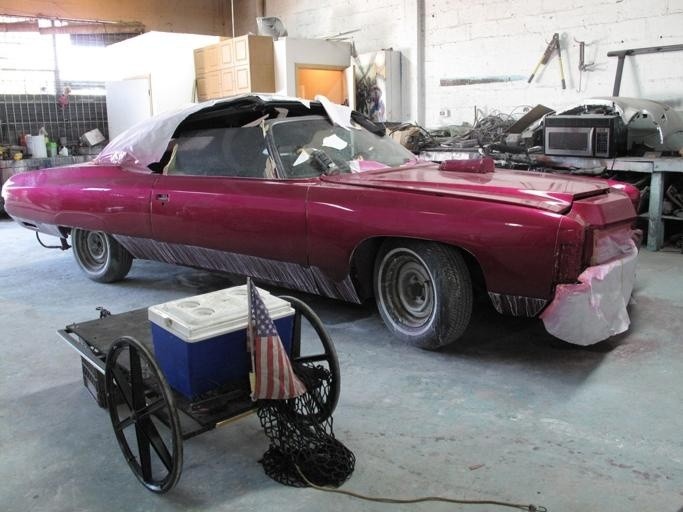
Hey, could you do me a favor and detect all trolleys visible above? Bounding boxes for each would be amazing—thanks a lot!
[51,282,347,497]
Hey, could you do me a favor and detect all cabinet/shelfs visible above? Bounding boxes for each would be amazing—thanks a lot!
[193,35,275,102]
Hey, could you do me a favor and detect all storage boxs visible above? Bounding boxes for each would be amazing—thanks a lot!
[148,285,296,401]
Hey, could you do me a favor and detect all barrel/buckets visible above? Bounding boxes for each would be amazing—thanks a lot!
[26,135,47,158]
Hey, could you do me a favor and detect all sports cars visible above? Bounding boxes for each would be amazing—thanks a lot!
[0,90,654,358]
